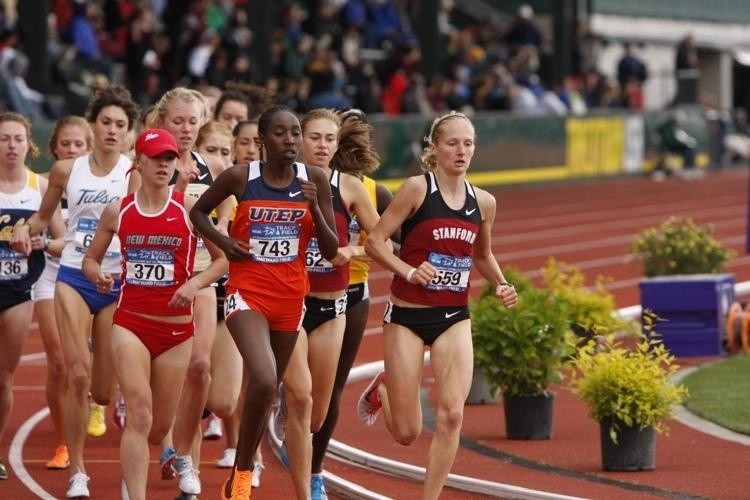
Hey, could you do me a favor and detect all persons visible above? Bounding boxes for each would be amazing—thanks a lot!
[1,1,650,126]
[670,36,701,109]
[652,113,699,181]
[1,79,517,499]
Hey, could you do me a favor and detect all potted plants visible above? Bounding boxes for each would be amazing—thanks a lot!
[469,268,577,439]
[560,307,688,472]
[465,294,501,402]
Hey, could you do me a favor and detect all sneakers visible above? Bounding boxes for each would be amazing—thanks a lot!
[310,472,328,500]
[173,491,198,500]
[0,461,8,479]
[159,448,176,480]
[216,448,237,468]
[112,394,126,430]
[204,416,223,438]
[170,455,202,494]
[279,443,289,468]
[85,402,107,437]
[121,480,130,500]
[356,370,386,426]
[221,465,251,500]
[45,444,70,468]
[251,459,266,487]
[65,472,91,499]
[273,382,287,441]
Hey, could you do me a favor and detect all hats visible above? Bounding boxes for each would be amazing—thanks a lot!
[134,128,180,159]
